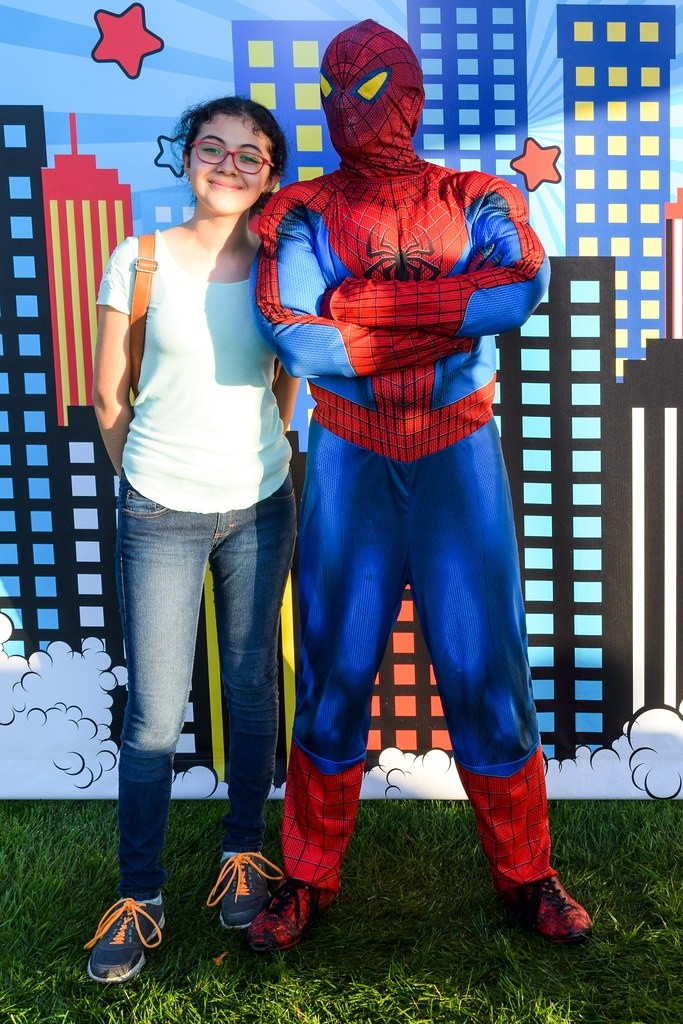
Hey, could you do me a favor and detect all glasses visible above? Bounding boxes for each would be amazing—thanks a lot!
[189,139,276,174]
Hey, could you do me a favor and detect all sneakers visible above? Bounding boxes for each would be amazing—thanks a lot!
[247,871,338,951]
[205,852,284,929]
[84,890,166,983]
[503,873,592,942]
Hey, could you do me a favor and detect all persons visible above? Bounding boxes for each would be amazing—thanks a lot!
[243,17,594,951]
[82,97,301,980]
